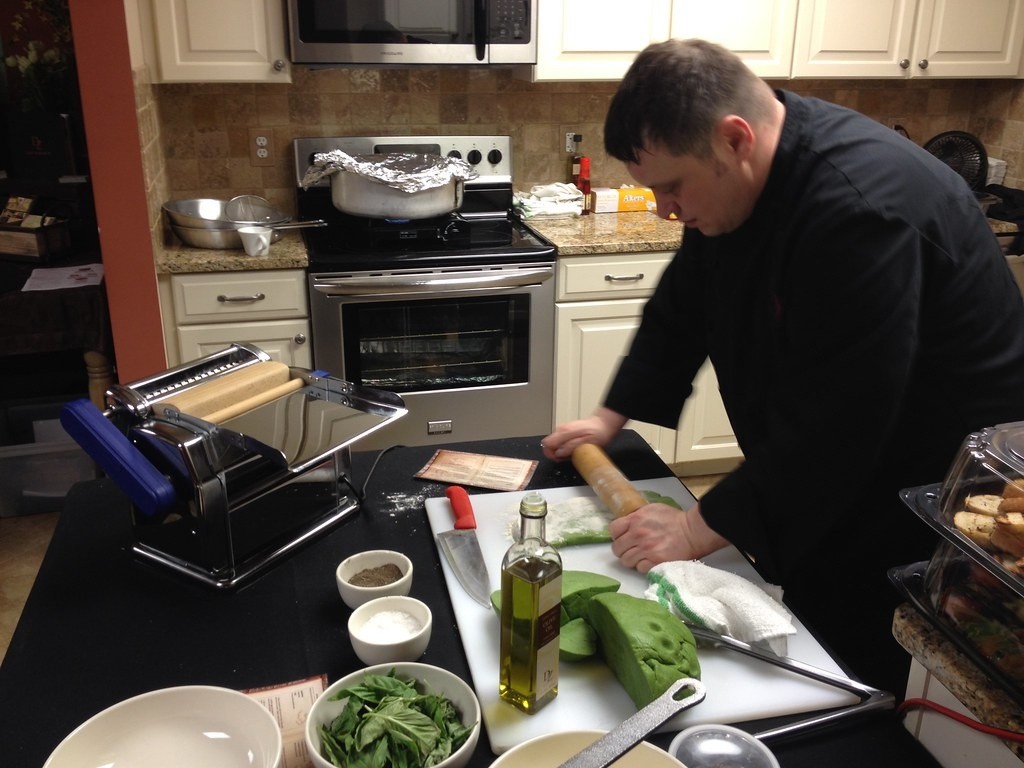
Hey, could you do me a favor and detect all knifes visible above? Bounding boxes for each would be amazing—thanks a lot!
[436,485,493,609]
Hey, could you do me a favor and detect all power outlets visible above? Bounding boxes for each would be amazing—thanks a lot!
[888,118,905,136]
[249,128,275,167]
[559,125,579,159]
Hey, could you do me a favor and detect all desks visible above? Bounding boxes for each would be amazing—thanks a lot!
[0,248,113,412]
[1,429,942,767]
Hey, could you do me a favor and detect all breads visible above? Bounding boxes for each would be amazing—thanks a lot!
[955,480,1024,582]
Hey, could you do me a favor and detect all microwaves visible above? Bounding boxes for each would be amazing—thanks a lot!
[285,0,539,72]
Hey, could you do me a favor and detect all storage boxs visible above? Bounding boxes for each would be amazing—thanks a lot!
[0,394,101,517]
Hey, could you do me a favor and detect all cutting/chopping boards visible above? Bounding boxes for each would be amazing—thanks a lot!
[423,475,864,754]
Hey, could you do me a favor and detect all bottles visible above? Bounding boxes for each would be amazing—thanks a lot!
[566,134,585,184]
[576,157,591,215]
[499,492,564,715]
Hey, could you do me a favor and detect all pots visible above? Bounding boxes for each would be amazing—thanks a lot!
[162,199,328,248]
[330,166,455,216]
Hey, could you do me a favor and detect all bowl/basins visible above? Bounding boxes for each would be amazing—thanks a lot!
[347,595,433,667]
[42,684,284,768]
[488,729,687,768]
[668,722,781,768]
[335,549,413,610]
[305,662,482,768]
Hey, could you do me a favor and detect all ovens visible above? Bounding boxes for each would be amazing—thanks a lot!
[307,262,555,453]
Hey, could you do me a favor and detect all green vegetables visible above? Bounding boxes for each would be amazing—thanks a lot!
[957,620,1021,661]
[320,667,478,768]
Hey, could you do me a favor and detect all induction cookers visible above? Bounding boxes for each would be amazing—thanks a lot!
[291,134,559,278]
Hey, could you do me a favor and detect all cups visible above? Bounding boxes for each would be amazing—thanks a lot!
[237,226,273,257]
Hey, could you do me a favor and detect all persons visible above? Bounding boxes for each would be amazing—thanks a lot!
[542,40,1023,706]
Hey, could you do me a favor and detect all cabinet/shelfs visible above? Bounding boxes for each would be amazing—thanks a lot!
[171,270,312,371]
[512,0,799,80]
[137,0,292,84]
[791,0,1024,79]
[552,250,745,479]
